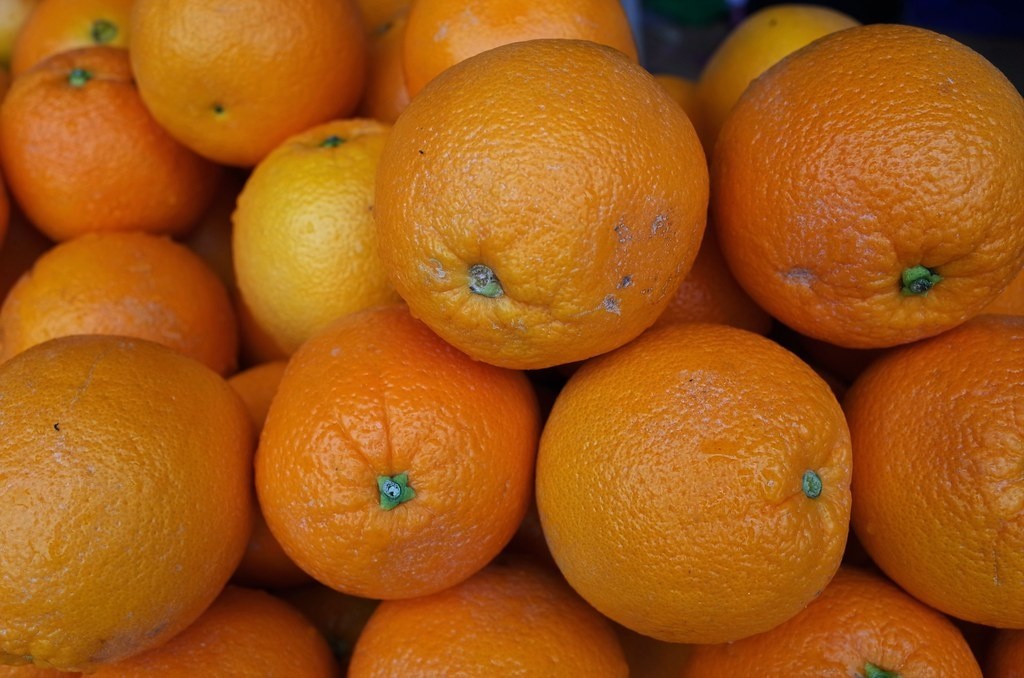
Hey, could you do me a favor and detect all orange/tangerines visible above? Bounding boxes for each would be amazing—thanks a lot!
[0,0,1024,678]
